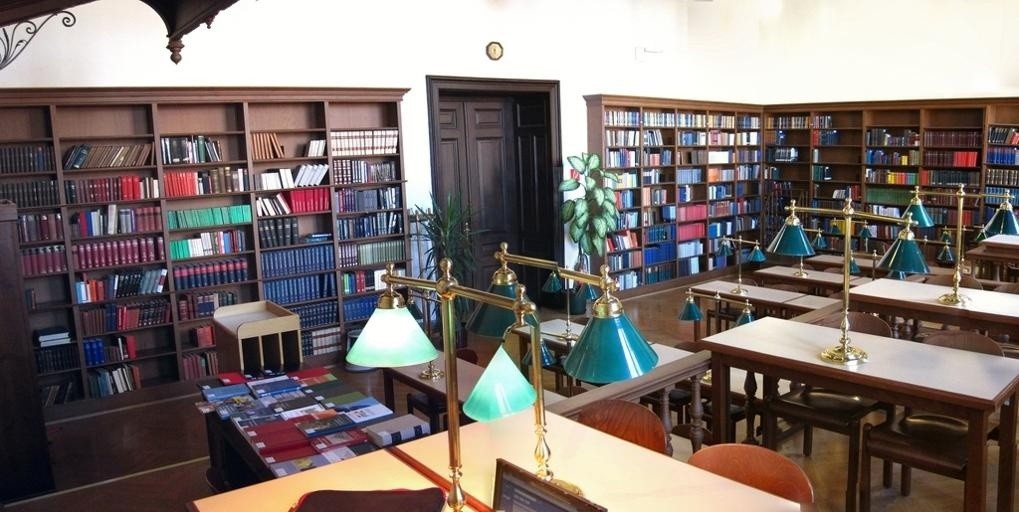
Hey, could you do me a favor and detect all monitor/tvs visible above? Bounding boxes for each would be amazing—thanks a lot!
[493,458,607,512]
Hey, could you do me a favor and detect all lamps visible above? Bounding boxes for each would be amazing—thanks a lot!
[466,243,659,384]
[764,183,931,364]
[716,235,766,295]
[899,183,1018,306]
[679,287,755,332]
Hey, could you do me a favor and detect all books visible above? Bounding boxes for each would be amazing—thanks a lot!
[865,125,1019,241]
[1,126,407,406]
[199,366,431,481]
[603,110,862,293]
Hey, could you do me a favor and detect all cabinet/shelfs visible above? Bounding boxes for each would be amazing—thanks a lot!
[1,88,416,425]
